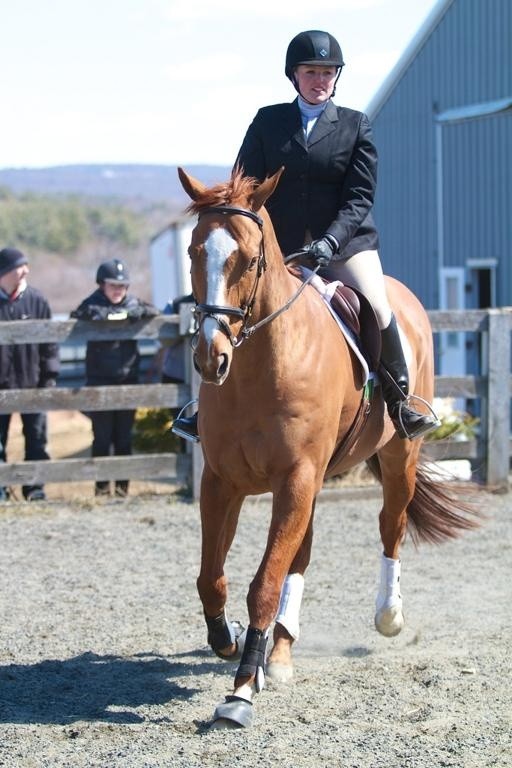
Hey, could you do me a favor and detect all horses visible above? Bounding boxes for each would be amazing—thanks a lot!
[177,156,497,732]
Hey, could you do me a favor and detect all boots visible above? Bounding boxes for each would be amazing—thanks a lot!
[173,411,198,436]
[378,312,430,440]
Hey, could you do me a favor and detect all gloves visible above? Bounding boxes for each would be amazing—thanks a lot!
[302,239,334,268]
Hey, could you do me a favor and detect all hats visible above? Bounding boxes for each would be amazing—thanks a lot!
[1,249,27,275]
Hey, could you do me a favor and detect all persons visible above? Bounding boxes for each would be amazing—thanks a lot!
[68,258,160,505]
[0,247,60,504]
[168,31,436,441]
[158,293,201,503]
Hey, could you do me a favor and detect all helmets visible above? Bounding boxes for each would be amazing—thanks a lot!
[97,261,130,288]
[286,31,344,78]
